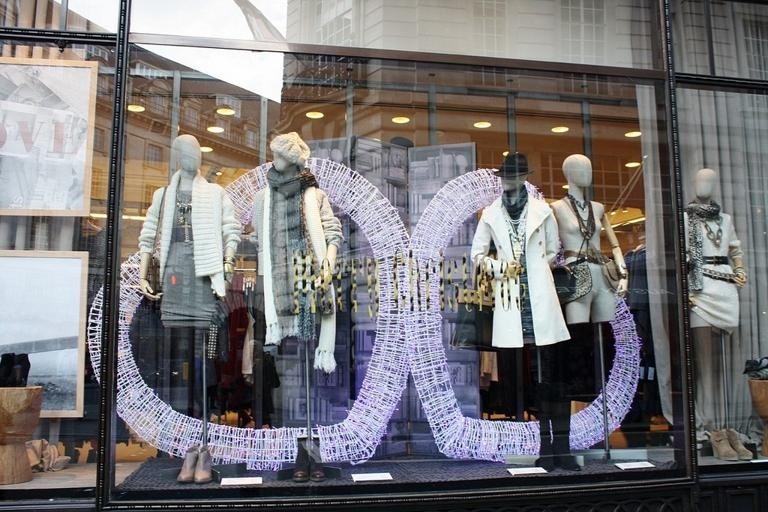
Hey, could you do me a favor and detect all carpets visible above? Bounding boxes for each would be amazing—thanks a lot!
[117,458,676,493]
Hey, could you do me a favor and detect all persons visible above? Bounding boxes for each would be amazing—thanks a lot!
[684,169,754,462]
[139,135,243,326]
[471,150,626,351]
[252,133,339,314]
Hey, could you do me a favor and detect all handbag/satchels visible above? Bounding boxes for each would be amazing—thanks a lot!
[602,259,618,292]
[552,258,592,305]
[147,258,160,296]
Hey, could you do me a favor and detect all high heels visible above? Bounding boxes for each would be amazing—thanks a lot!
[710,428,753,461]
[1,353,30,386]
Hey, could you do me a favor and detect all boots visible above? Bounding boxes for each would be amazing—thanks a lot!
[535,383,580,471]
[294,437,325,482]
[177,447,212,482]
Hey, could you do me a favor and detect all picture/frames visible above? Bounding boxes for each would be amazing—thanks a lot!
[0,56,100,217]
[2,249,90,420]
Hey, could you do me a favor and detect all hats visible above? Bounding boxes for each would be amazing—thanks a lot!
[269,132,310,165]
[493,152,534,178]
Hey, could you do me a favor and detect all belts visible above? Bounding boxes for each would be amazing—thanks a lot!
[702,256,728,264]
[565,250,595,261]
